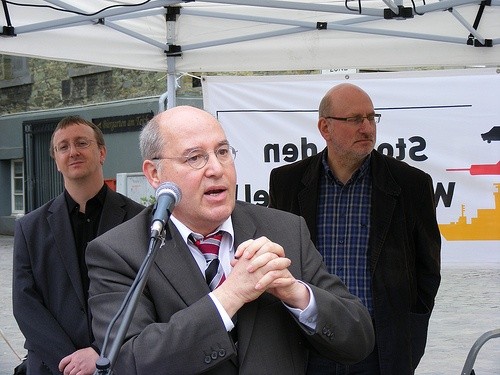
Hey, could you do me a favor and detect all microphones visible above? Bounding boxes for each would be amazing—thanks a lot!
[149,182,182,242]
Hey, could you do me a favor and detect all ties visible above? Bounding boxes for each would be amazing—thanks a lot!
[187,230,226,292]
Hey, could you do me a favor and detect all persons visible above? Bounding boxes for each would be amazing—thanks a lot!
[268,83,442,375]
[12,116,147,375]
[85,105,375,374]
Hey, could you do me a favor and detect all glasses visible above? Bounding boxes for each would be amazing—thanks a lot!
[53,139,105,153]
[151,144,238,169]
[324,114,382,125]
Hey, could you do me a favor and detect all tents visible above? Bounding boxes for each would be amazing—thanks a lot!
[0,0,499,110]
